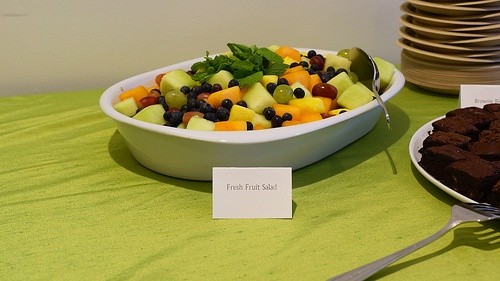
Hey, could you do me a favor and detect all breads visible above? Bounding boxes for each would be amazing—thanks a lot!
[417,104,500,206]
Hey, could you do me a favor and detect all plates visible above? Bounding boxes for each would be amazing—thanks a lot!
[100,47,405,181]
[408,106,499,220]
[395,1,500,94]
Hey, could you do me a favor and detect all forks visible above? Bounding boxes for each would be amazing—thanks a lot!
[326,202,500,281]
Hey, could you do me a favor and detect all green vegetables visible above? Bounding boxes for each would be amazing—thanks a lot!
[193,42,289,90]
